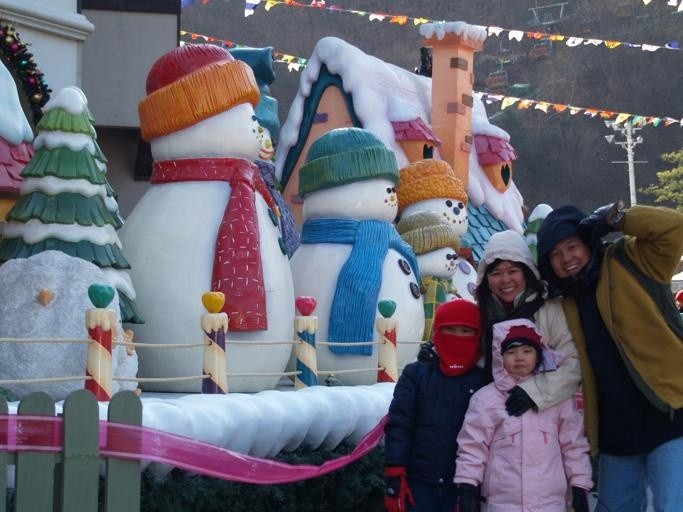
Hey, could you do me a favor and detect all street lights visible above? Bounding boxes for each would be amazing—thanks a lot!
[601,117,648,207]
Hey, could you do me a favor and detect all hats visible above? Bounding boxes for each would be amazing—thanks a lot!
[501,325,542,356]
[430,300,482,377]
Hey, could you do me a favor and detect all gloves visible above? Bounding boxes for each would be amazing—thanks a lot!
[505,385,534,416]
[385,467,415,512]
[458,483,481,512]
[571,486,589,512]
[579,202,625,236]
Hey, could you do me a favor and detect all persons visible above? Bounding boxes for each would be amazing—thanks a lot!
[674,290,683,319]
[417,230,582,416]
[455,319,593,512]
[537,201,683,511]
[383,299,494,512]
